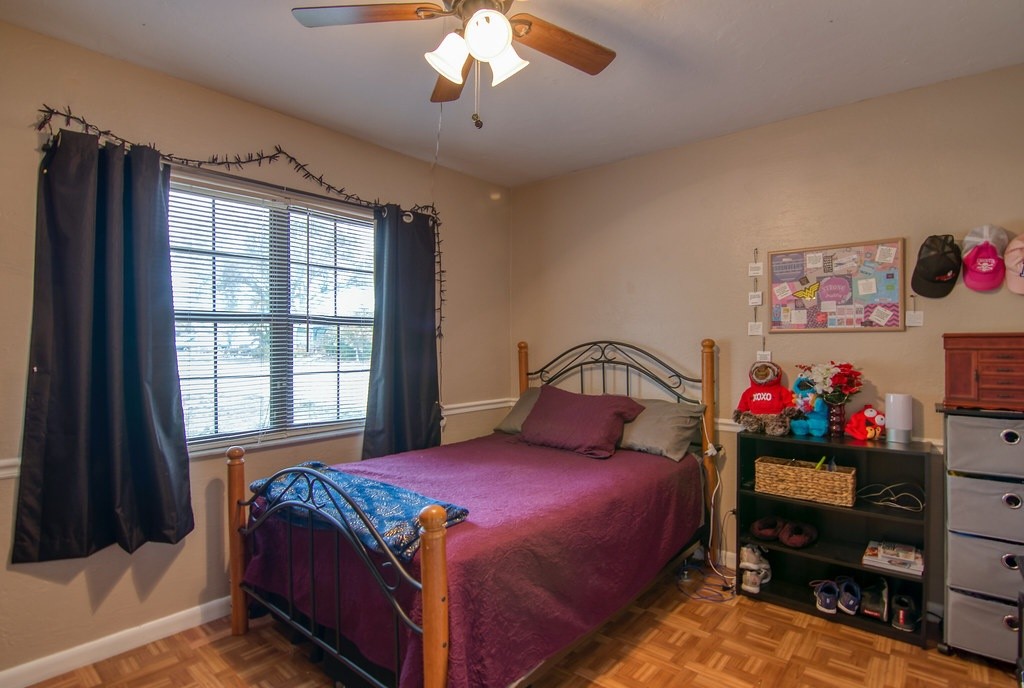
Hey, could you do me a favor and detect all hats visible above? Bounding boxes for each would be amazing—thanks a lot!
[911,234,961,298]
[961,224,1008,290]
[1003,235,1024,294]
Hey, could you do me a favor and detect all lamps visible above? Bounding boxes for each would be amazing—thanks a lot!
[424,0,530,129]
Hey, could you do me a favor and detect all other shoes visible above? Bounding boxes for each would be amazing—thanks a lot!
[752,518,784,540]
[779,521,818,548]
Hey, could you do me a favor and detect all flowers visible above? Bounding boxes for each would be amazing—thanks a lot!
[794,360,864,405]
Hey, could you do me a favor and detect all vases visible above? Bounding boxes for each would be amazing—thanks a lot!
[824,395,850,438]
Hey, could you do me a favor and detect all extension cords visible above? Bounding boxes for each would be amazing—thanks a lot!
[708,443,717,456]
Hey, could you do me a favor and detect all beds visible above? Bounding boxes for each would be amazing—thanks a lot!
[227,338,719,688]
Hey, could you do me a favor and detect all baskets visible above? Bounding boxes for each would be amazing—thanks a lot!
[754,457,855,508]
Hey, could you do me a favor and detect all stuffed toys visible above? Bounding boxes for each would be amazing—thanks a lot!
[845,405,886,440]
[731,361,796,435]
[790,372,829,435]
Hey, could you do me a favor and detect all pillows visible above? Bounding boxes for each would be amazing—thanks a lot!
[617,398,707,462]
[494,387,540,435]
[506,384,645,459]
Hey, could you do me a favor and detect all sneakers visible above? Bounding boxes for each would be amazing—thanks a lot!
[835,573,860,615]
[741,572,761,594]
[809,579,839,613]
[740,546,770,570]
[890,593,916,632]
[860,577,889,622]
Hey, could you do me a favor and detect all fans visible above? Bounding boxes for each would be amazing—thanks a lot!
[291,0,616,102]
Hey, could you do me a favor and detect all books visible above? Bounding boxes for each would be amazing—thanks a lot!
[862,540,924,575]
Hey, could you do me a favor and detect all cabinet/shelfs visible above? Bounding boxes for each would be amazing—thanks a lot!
[941,332,1024,412]
[735,428,933,651]
[934,402,1024,685]
